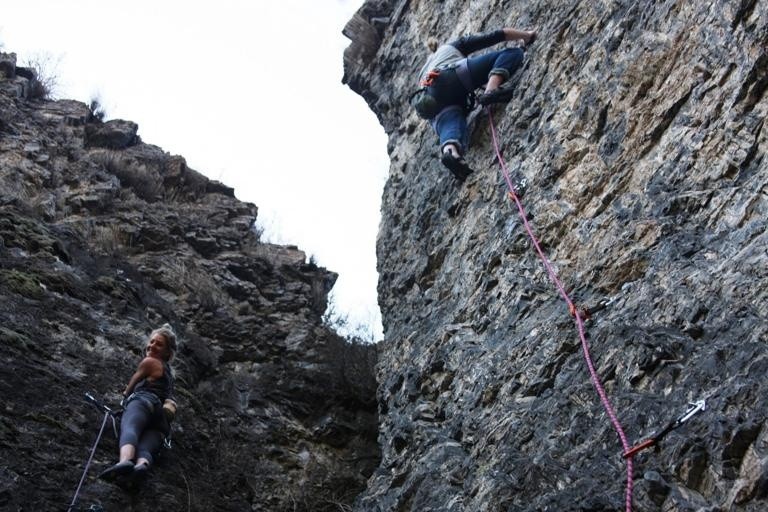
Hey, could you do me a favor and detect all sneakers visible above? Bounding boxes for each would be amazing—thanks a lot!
[477,86,515,106]
[440,147,473,181]
[121,461,149,485]
[98,458,135,482]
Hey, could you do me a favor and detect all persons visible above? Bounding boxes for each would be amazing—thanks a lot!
[417,25,540,181]
[97,322,178,488]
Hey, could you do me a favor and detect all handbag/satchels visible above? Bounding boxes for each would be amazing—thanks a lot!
[415,95,437,120]
